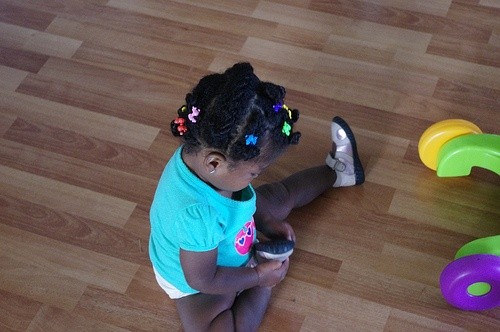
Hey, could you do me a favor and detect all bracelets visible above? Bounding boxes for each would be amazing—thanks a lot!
[253,265,262,288]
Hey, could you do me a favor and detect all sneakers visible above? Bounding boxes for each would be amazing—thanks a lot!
[327,117,366,188]
[255,239,294,264]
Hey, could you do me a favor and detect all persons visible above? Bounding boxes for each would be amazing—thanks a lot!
[148,60,365,332]
